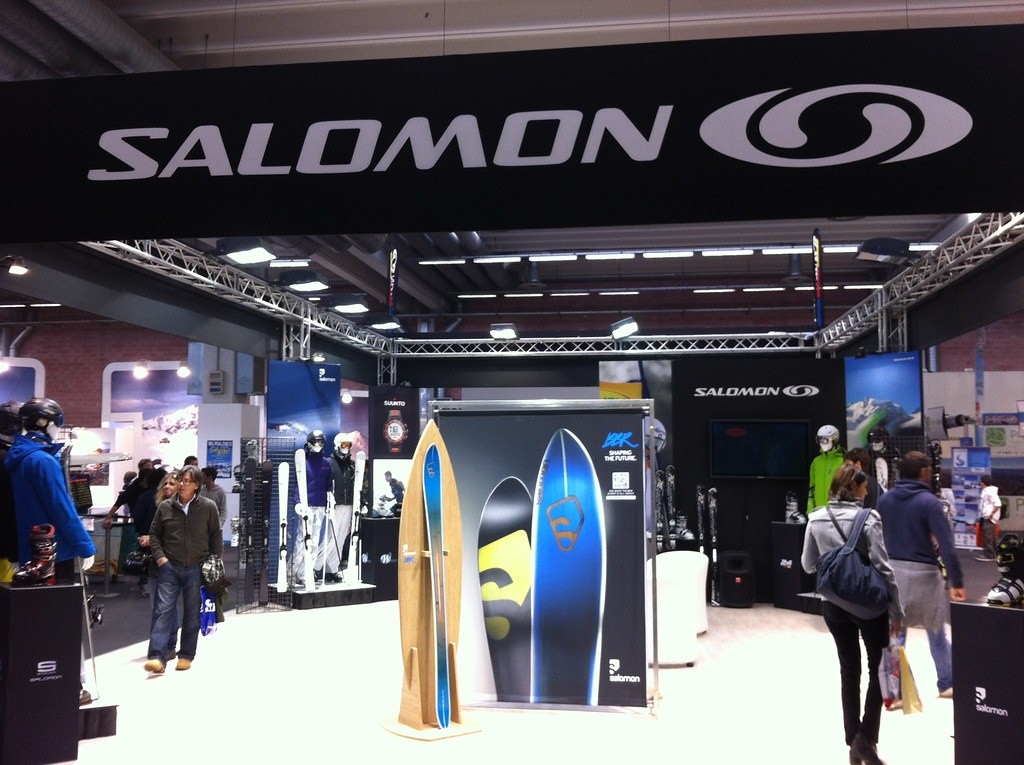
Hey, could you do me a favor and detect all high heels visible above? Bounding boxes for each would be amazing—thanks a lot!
[849,733,887,765]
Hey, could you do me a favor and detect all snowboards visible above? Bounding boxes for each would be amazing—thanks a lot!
[476,475,534,702]
[532,426,607,705]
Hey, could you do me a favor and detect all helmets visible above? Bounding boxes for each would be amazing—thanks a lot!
[0,400,24,434]
[867,427,889,440]
[818,425,840,439]
[20,397,64,431]
[334,432,354,444]
[306,430,326,443]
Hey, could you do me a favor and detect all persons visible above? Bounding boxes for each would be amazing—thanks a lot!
[383,469,406,516]
[286,429,333,588]
[873,452,967,704]
[806,425,850,515]
[313,431,355,582]
[0,396,96,585]
[939,471,958,527]
[845,446,884,510]
[798,463,908,765]
[100,453,227,672]
[865,427,903,493]
[975,475,1001,561]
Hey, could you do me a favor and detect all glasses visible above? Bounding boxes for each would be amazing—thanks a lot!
[342,441,353,449]
[816,436,830,444]
[175,478,197,486]
[868,436,883,444]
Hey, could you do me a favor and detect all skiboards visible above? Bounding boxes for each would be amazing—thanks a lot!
[655,463,676,554]
[421,443,452,730]
[241,456,273,606]
[695,484,721,607]
[888,439,944,501]
[276,449,316,594]
[345,449,367,584]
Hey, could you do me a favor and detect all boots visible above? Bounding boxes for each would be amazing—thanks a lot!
[12,523,57,586]
[785,493,807,524]
[675,514,694,540]
[986,533,1024,604]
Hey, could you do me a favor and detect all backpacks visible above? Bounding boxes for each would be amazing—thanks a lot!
[999,497,1010,520]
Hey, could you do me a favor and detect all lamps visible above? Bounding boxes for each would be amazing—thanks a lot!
[313,351,352,406]
[207,237,942,341]
[0,254,27,275]
[134,356,190,380]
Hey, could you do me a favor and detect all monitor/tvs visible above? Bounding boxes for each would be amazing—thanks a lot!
[708,418,811,479]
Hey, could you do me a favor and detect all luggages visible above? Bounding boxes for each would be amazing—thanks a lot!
[717,549,756,608]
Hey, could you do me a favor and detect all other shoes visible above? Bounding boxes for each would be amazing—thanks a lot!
[313,568,344,582]
[974,552,997,561]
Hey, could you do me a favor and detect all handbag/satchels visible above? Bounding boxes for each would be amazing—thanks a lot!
[815,508,898,620]
[878,632,924,715]
[121,543,148,571]
[200,585,216,636]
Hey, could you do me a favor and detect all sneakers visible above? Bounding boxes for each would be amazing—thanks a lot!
[144,659,165,673]
[176,658,191,670]
